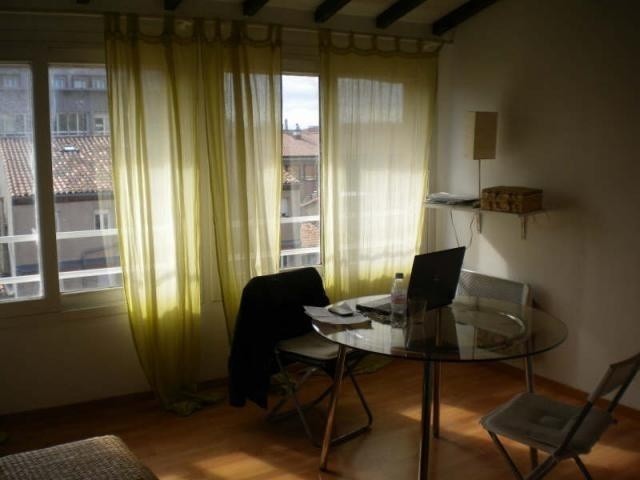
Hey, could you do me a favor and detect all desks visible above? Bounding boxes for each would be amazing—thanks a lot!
[312,294,567,480]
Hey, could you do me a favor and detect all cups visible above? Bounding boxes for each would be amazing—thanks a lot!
[409,296,428,324]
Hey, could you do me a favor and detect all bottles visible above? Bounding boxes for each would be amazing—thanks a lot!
[390,272,409,328]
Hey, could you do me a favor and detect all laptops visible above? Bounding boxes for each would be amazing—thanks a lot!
[355,246,465,320]
[363,307,462,360]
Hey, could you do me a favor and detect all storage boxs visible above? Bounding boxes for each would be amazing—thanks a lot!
[481,186,543,213]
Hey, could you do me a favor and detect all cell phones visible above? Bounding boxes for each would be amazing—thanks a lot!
[328,307,353,317]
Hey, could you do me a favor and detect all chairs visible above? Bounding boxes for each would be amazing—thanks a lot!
[250,267,373,450]
[478,353,640,480]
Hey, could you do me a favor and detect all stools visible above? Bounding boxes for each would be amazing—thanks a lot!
[0,434,159,480]
